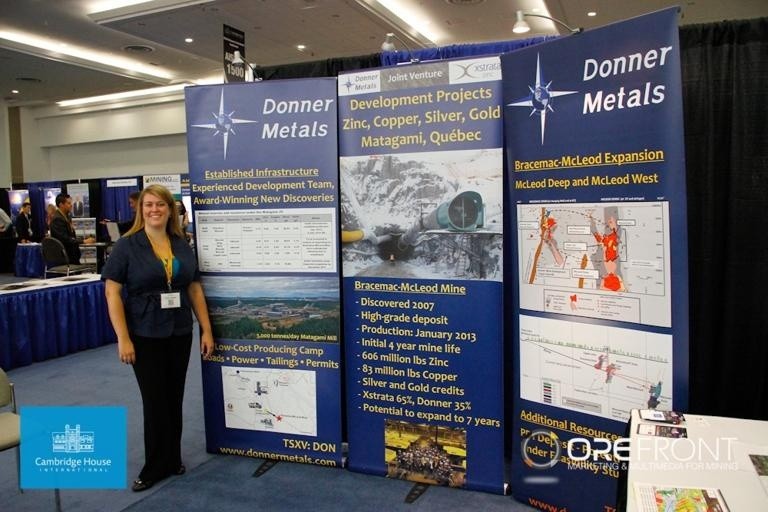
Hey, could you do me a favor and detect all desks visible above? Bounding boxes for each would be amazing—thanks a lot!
[14,242,43,278]
[0,272,119,371]
[78,242,111,273]
[624,408,768,512]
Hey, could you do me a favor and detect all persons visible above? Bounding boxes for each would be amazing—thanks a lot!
[0,208,13,233]
[102,183,215,494]
[174,200,190,244]
[46,204,56,230]
[16,203,39,277]
[50,193,96,276]
[99,191,141,236]
[73,192,84,216]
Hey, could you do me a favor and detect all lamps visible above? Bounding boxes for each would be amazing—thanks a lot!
[512,10,583,36]
[231,49,263,83]
[380,32,420,64]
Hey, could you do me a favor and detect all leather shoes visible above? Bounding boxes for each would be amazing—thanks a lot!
[131,464,187,492]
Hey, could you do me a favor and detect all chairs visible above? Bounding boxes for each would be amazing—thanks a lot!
[42,237,95,280]
[0,368,61,512]
